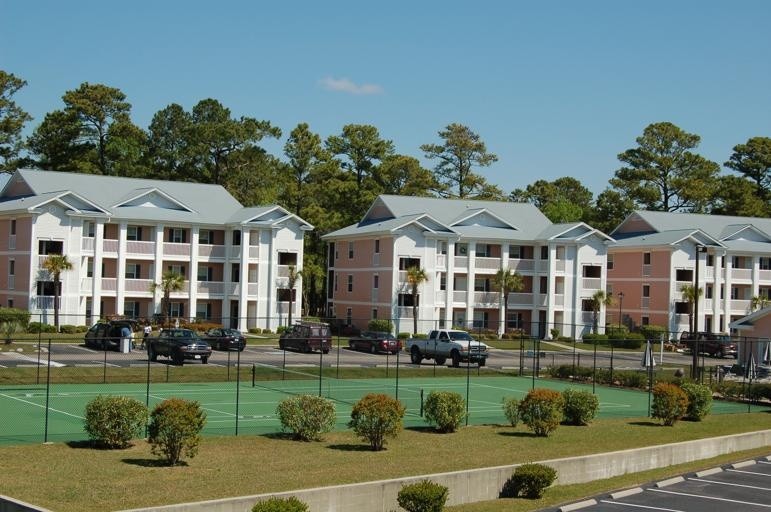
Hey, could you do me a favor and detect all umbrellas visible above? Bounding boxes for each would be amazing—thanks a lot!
[641,340,657,375]
[764,339,771,366]
[747,353,758,382]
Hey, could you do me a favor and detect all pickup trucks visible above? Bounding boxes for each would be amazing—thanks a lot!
[144,326,212,365]
[404,330,488,368]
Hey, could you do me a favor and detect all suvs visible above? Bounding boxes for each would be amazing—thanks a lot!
[83,320,139,352]
[680,329,738,359]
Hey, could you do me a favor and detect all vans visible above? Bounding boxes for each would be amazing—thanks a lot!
[280,319,331,354]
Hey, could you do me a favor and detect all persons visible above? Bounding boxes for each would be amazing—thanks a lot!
[141,320,153,350]
[121,324,132,354]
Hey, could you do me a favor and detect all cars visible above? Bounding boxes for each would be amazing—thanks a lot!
[347,330,400,355]
[198,326,246,352]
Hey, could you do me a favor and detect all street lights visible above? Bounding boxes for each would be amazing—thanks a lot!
[616,290,624,333]
[690,243,726,381]
